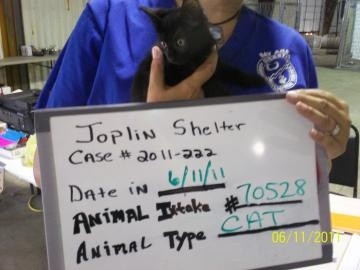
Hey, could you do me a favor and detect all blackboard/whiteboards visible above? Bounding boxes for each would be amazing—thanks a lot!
[32,89,336,270]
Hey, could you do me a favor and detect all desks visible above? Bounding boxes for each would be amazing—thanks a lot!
[0,50,63,86]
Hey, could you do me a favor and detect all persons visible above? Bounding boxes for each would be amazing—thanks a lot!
[26,0,351,191]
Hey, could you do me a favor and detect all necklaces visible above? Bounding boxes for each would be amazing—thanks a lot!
[204,9,240,42]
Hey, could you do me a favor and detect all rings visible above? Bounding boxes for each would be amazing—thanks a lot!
[330,124,341,137]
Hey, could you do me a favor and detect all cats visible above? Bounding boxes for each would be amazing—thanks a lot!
[131,0,267,102]
